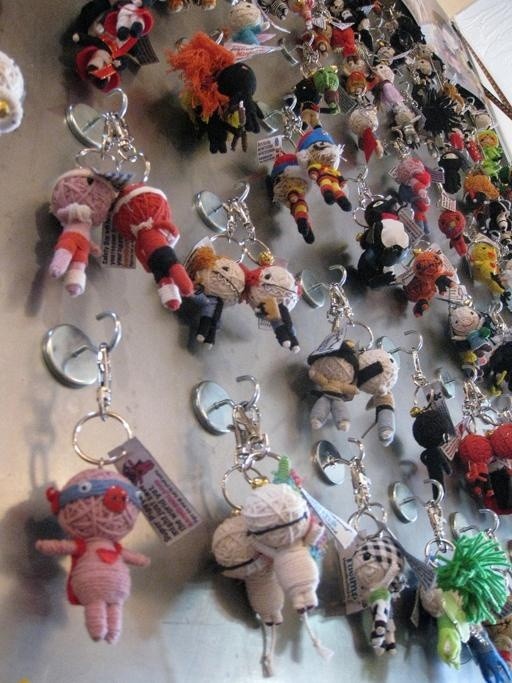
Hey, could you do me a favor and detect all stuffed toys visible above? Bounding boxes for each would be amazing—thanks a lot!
[1,1,510,682]
[0,0,510,683]
